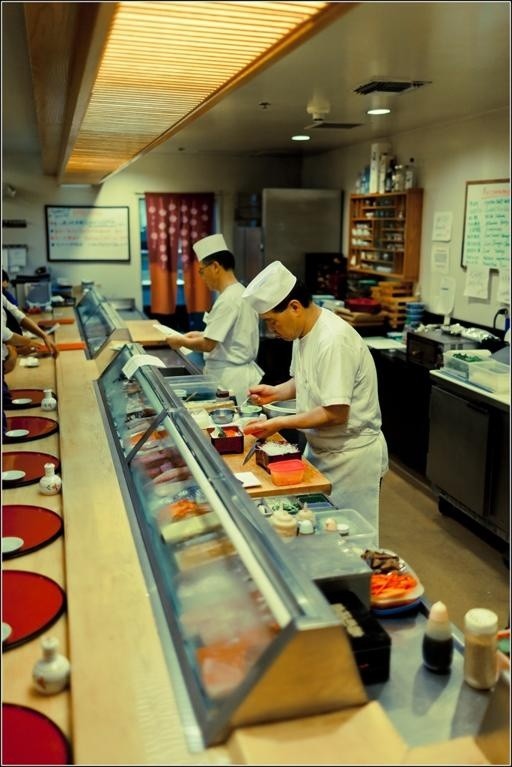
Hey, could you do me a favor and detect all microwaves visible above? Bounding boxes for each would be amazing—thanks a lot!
[406,331,478,371]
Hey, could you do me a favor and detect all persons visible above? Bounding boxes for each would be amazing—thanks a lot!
[3,269,21,307]
[243,278,389,548]
[15,341,41,355]
[1,343,18,434]
[165,249,265,402]
[2,292,60,358]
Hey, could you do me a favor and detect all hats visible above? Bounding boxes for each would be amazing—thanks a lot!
[192,232,240,263]
[240,259,300,315]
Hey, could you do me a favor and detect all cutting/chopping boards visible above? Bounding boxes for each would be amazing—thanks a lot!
[125,319,168,346]
[201,419,332,498]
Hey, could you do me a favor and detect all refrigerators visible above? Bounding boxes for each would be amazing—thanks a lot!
[233,188,344,287]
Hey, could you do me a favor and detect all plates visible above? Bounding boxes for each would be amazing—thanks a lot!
[2,620,12,643]
[2,536,24,553]
[232,471,261,489]
[12,398,32,406]
[357,548,424,616]
[5,429,30,438]
[2,470,26,481]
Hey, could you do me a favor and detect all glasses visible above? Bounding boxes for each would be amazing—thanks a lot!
[198,259,215,273]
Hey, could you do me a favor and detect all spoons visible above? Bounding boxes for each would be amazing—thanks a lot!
[232,395,251,408]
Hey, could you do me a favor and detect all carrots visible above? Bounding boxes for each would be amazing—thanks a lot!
[372,571,416,596]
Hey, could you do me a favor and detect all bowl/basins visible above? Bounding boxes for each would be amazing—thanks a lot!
[405,302,425,326]
[236,405,263,418]
[387,331,404,342]
[345,298,381,315]
[209,408,236,424]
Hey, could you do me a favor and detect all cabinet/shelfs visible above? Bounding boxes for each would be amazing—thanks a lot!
[345,187,423,282]
[426,384,509,569]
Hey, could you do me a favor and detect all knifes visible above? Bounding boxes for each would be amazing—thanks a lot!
[243,437,266,466]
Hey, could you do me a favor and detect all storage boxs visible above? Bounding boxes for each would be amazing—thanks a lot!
[442,350,510,393]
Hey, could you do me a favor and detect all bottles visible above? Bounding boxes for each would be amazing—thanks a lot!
[384,155,416,192]
[269,501,349,538]
[39,463,62,496]
[215,391,229,403]
[31,636,69,696]
[355,172,365,194]
[41,389,57,411]
[462,607,500,691]
[421,600,455,674]
